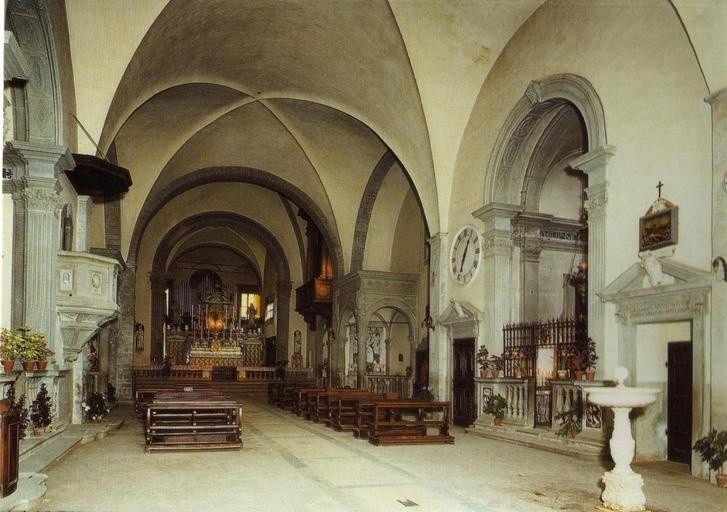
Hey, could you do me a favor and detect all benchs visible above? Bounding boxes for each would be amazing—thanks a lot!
[135,381,243,454]
[267,380,457,446]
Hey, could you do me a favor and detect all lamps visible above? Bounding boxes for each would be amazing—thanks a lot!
[134,322,144,352]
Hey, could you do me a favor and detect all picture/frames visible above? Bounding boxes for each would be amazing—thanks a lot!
[637,207,677,251]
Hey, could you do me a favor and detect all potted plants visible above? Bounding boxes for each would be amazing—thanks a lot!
[476,337,600,383]
[693,427,726,489]
[1,327,52,372]
[1,381,117,439]
[483,392,507,426]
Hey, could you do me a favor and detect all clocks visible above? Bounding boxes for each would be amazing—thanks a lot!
[447,224,482,287]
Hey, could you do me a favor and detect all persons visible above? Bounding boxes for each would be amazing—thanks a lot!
[246,304,258,335]
[171,300,183,332]
[136,325,144,352]
[294,329,301,354]
